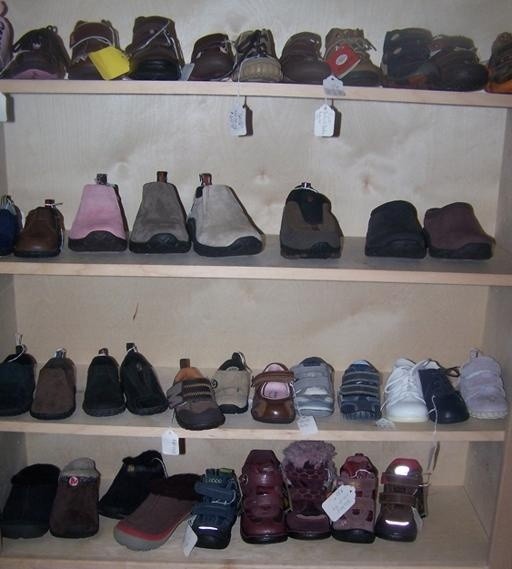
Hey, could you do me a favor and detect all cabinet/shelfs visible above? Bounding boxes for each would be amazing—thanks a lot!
[0,78,512,569]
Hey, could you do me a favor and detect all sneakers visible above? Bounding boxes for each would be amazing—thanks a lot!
[383,29,432,93]
[132,169,192,257]
[66,169,130,257]
[236,29,279,81]
[323,26,382,86]
[195,171,264,257]
[0,18,70,82]
[69,16,124,80]
[432,31,487,92]
[279,181,343,260]
[488,31,511,92]
[1,440,430,550]
[365,198,423,260]
[0,338,510,431]
[281,28,330,81]
[423,202,498,262]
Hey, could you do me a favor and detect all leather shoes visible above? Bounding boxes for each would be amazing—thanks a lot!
[189,34,235,82]
[129,17,185,80]
[0,193,65,259]
